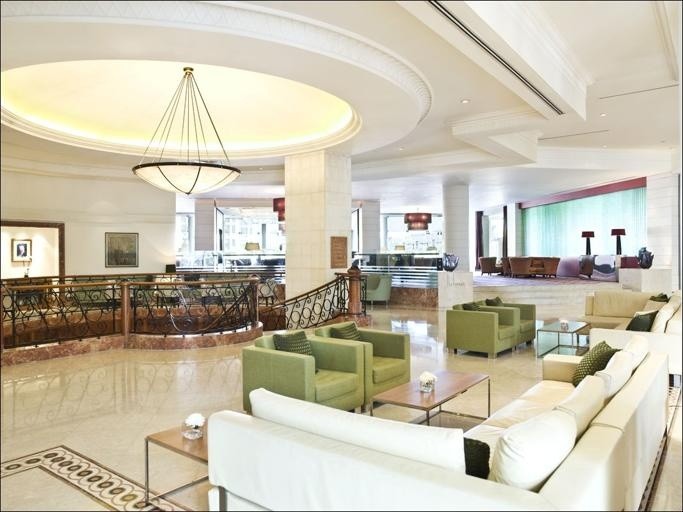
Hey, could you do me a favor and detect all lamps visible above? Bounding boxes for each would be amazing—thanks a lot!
[133,67,241,196]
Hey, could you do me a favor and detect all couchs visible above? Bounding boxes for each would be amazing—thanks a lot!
[208,350,670,511]
[361,275,392,304]
[446,305,520,359]
[530,256,560,278]
[478,256,503,276]
[585,289,682,375]
[501,257,512,277]
[315,321,410,412]
[481,298,535,350]
[242,328,365,412]
[509,257,536,278]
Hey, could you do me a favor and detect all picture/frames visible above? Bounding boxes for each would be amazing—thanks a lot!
[11,239,32,262]
[105,232,139,268]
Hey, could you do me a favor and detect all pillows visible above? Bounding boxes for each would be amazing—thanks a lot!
[486,297,503,307]
[273,332,317,373]
[249,388,464,471]
[597,352,634,392]
[464,437,490,480]
[626,311,657,332]
[330,323,361,340]
[490,410,578,490]
[572,342,621,387]
[463,302,481,310]
[643,293,670,311]
[555,376,608,438]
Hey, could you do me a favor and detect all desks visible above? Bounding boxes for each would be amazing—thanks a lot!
[373,371,490,426]
[144,418,208,510]
[537,322,590,358]
